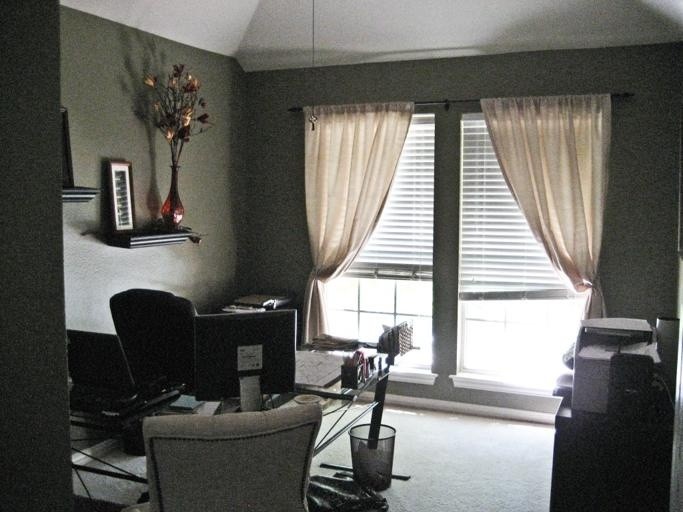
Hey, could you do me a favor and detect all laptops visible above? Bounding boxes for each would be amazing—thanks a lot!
[65,330,185,392]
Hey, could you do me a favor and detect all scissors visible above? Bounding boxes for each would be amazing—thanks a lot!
[345,356,355,367]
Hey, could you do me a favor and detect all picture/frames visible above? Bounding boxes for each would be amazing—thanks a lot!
[108,160,135,232]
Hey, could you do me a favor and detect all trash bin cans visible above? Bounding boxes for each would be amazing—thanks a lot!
[348,423,397,492]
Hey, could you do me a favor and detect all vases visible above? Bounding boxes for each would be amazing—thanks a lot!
[160,166,184,226]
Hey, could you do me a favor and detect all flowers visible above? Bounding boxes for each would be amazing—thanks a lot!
[139,65,216,212]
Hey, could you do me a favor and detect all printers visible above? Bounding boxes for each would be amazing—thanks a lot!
[552,318,652,397]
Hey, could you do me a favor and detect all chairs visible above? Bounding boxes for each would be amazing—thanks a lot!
[142,402,321,512]
[108,289,201,392]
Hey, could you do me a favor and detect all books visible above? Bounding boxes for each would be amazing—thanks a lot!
[295,349,350,388]
[162,398,222,416]
[169,393,206,415]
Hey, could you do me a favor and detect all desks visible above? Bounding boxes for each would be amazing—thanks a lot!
[288,334,420,446]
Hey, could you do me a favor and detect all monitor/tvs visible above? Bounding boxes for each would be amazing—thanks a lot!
[192,309,298,412]
